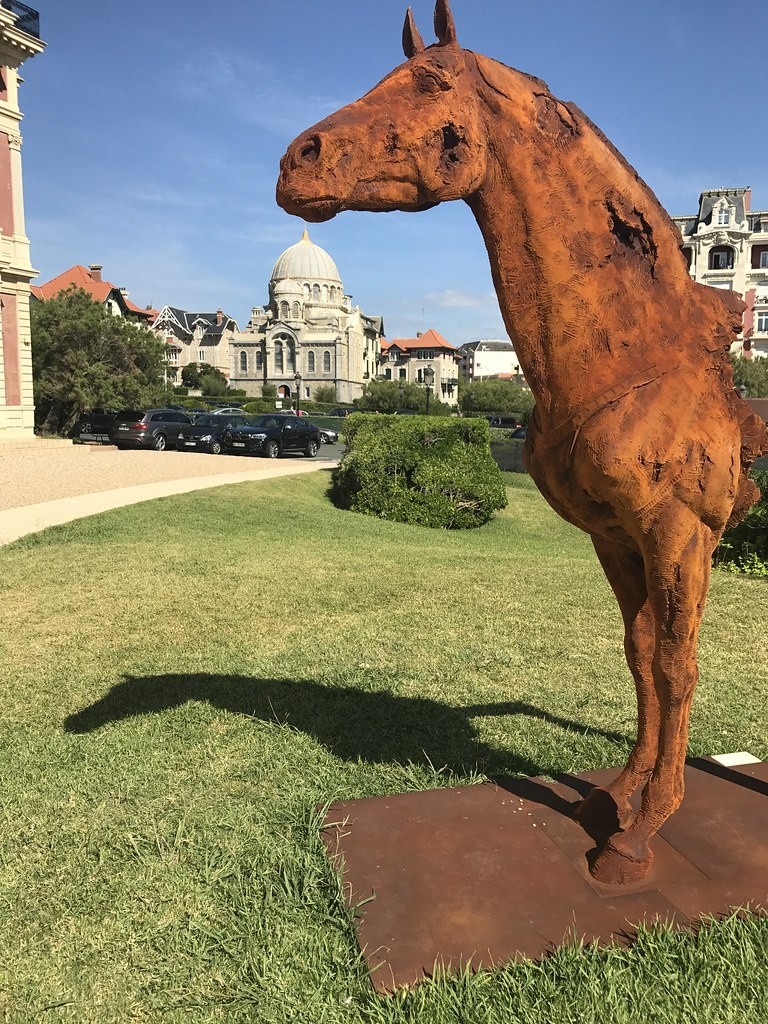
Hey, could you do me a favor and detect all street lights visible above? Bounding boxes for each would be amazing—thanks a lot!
[423,364,435,414]
[164,328,176,391]
[294,371,302,416]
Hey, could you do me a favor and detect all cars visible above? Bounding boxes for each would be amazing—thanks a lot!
[176,414,248,454]
[326,407,354,417]
[225,413,321,457]
[511,427,528,438]
[210,408,245,414]
[319,427,338,444]
[79,408,118,433]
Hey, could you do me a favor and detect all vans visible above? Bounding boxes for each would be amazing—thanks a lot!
[486,414,516,429]
[281,410,308,416]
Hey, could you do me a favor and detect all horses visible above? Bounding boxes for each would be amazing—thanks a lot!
[276,1,768,884]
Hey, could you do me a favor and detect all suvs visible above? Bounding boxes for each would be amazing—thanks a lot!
[108,409,194,451]
